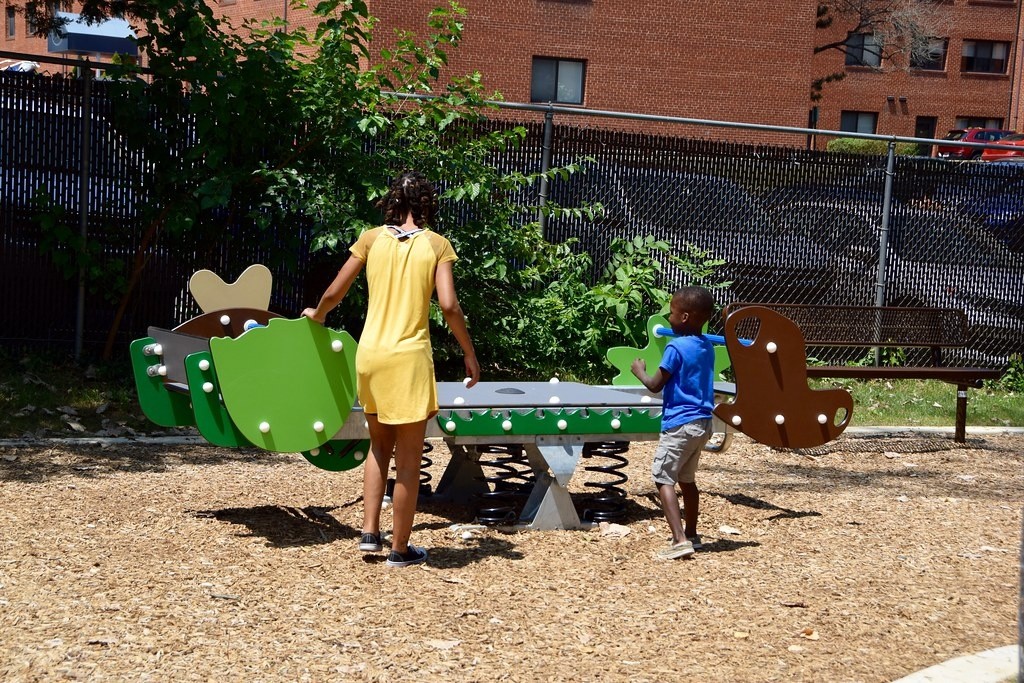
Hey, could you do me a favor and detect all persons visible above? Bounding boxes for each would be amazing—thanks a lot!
[629,286,717,558]
[301,170,480,566]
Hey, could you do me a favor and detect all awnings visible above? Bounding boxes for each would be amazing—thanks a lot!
[46,11,139,77]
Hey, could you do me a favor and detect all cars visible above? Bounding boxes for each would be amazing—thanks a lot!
[938,128,1017,161]
[0,96,190,296]
[768,202,1024,368]
[981,133,1024,162]
[829,155,947,202]
[544,162,841,318]
[932,161,1024,255]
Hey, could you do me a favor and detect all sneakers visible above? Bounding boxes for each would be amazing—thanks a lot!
[658,541,695,558]
[359,529,383,551]
[386,543,427,566]
[687,534,703,548]
